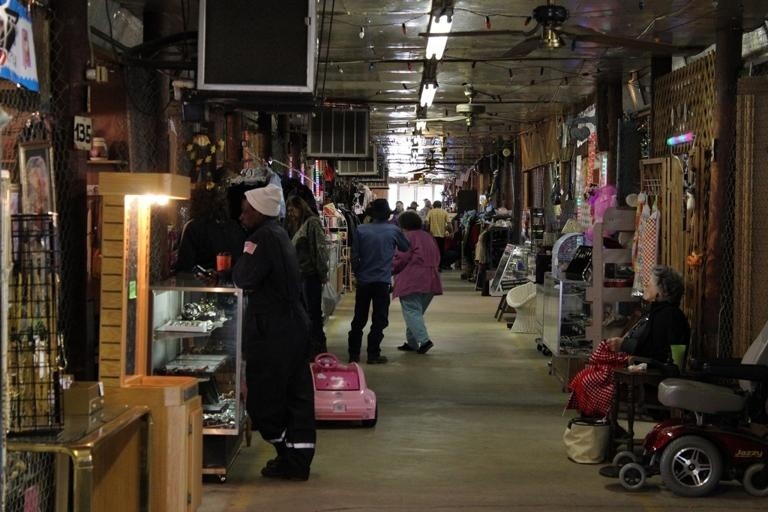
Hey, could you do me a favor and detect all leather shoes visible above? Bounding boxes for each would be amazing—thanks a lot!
[268,456,288,468]
[261,460,309,481]
[367,356,389,364]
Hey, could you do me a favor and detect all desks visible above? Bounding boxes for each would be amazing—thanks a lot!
[0,403,148,512]
[605,369,668,460]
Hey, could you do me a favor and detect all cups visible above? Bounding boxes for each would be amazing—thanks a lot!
[671,344,686,370]
[216,254,231,273]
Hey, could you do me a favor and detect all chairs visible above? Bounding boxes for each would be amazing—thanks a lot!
[657,319,767,418]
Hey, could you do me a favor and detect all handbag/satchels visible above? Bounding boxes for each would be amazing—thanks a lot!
[563,413,612,465]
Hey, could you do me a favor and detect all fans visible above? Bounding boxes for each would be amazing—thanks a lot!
[416,0,707,68]
[412,84,535,131]
[409,151,454,178]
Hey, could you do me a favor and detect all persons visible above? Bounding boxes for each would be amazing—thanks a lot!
[233,184,316,480]
[566,264,689,420]
[284,195,328,356]
[392,211,443,354]
[177,177,464,274]
[349,199,409,365]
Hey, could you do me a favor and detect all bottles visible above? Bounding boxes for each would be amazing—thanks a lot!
[89,137,108,160]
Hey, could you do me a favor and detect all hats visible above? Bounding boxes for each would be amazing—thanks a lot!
[367,198,397,221]
[244,181,285,218]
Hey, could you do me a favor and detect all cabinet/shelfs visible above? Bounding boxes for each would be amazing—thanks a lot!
[146,272,250,484]
[88,170,203,512]
[321,203,355,299]
[481,200,647,390]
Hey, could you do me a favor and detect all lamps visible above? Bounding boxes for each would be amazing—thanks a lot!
[537,19,563,50]
[421,0,457,69]
[416,70,438,109]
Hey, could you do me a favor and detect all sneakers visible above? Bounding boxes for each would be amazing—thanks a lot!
[398,342,412,351]
[417,340,433,354]
